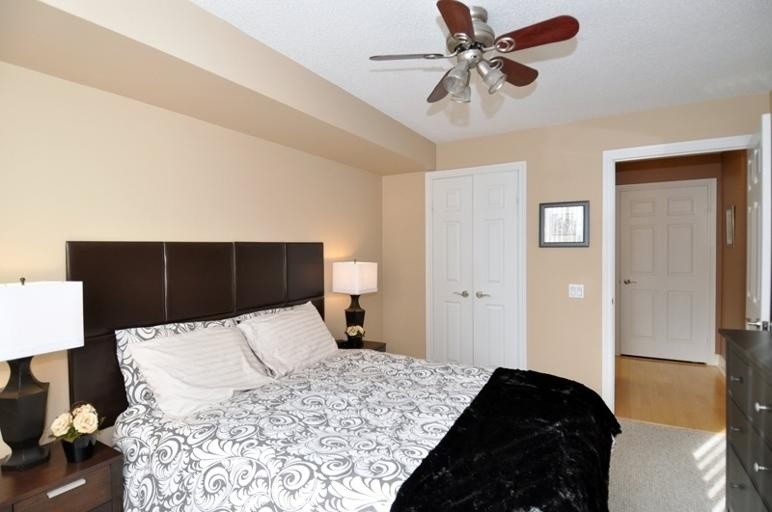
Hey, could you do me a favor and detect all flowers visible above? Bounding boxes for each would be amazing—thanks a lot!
[344,325,366,339]
[50,403,99,443]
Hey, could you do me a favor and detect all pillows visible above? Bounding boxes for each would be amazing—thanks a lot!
[112,300,340,423]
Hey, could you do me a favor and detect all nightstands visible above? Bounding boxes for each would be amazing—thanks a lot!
[0,437,126,512]
[336,339,386,353]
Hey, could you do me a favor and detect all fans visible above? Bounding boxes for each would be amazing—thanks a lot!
[369,0,580,103]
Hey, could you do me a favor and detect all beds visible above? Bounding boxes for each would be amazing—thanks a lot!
[64,239,623,512]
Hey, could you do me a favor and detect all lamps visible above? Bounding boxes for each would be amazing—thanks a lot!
[0,281,85,471]
[442,49,507,103]
[332,261,378,347]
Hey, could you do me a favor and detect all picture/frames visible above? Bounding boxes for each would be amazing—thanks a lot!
[539,200,590,248]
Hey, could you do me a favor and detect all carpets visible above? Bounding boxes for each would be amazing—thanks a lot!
[606,411,726,512]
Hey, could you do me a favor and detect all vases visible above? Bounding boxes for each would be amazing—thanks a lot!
[60,433,96,464]
[347,336,362,349]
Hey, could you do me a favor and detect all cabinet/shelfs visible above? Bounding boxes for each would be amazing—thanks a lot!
[716,328,772,512]
